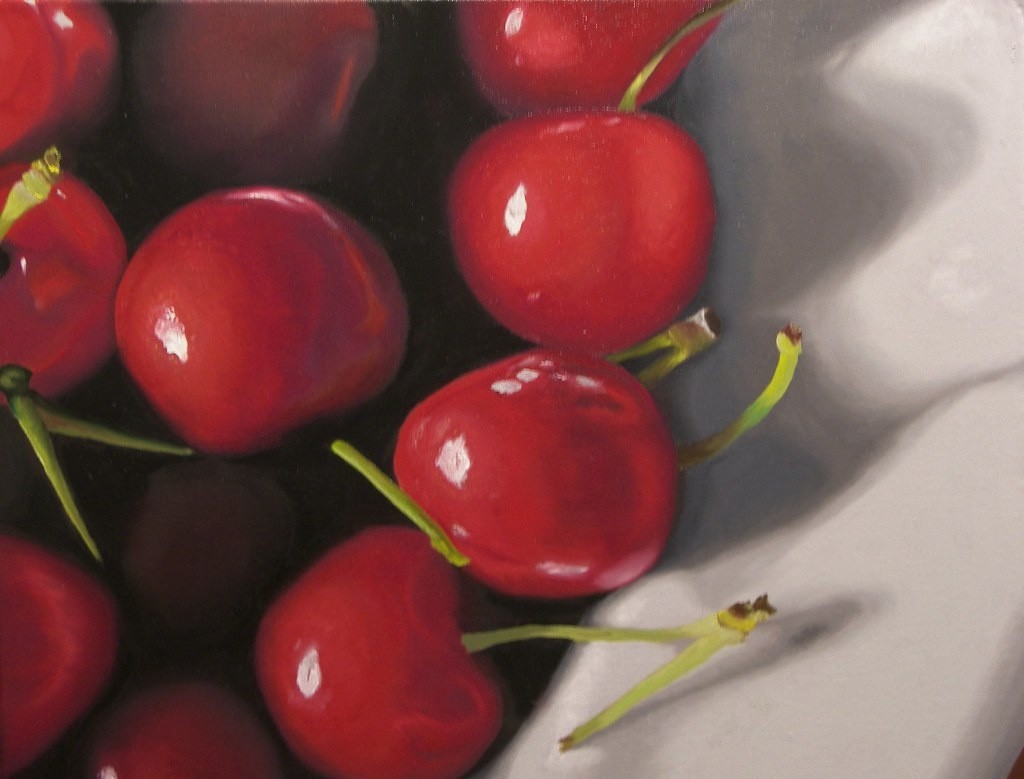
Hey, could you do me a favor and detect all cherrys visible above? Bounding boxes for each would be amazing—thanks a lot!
[0,0,805,779]
